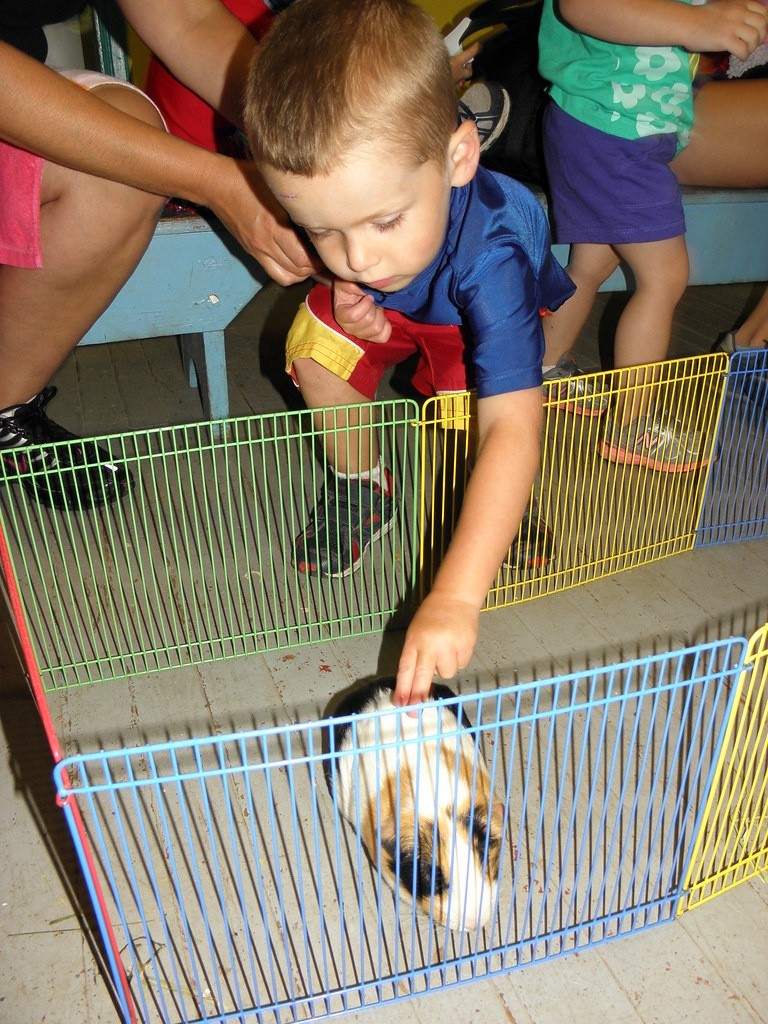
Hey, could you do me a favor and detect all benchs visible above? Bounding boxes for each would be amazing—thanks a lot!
[81,178,768,435]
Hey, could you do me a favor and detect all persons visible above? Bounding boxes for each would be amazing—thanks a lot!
[242,0,579,718]
[0,0,314,513]
[141,0,510,158]
[666,46,768,407]
[536,0,768,474]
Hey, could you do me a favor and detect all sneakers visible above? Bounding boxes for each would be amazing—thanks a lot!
[454,78,510,157]
[0,386,136,510]
[597,402,721,472]
[699,330,768,408]
[290,466,400,578]
[435,456,557,571]
[540,351,615,416]
